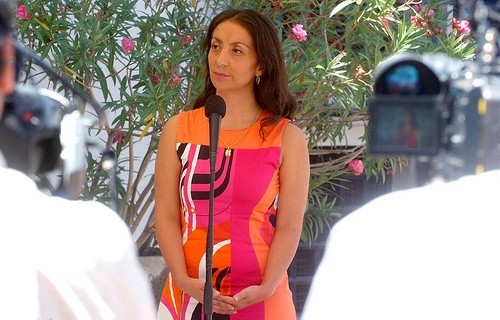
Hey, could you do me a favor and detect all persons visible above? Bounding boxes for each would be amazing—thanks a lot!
[300,167,500,320]
[155,8,310,320]
[0,0,158,320]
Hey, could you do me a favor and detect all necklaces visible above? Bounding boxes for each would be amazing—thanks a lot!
[217,105,260,156]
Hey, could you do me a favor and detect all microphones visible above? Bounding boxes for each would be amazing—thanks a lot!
[205,95,226,171]
